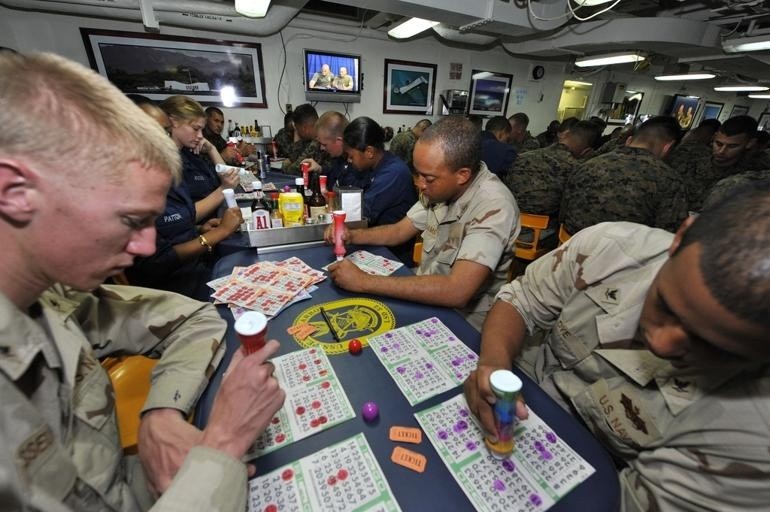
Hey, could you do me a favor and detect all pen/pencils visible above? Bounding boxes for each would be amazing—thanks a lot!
[321,307,340,342]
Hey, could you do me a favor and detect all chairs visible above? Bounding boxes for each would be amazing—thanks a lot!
[100,355,195,447]
[558,223,571,247]
[511,212,549,260]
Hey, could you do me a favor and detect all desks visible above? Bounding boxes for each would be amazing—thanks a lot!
[213,243,622,512]
[217,156,369,249]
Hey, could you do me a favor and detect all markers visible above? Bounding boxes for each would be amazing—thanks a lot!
[303,162,310,189]
[222,189,241,233]
[319,175,327,196]
[272,141,277,158]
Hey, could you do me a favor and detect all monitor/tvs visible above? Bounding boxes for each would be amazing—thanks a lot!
[668,94,702,131]
[303,47,361,104]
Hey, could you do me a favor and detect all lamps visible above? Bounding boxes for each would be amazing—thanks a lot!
[387,15,440,39]
[721,35,770,53]
[713,84,770,92]
[748,93,770,99]
[575,51,647,67]
[655,71,716,81]
[235,0,271,18]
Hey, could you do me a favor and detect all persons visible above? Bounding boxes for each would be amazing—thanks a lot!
[119,94,245,296]
[309,64,333,89]
[465,171,770,511]
[332,117,418,268]
[324,118,522,334]
[389,119,432,174]
[477,103,769,282]
[275,103,373,194]
[332,67,354,91]
[0,51,286,512]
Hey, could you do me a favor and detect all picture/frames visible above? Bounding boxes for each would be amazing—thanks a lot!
[383,58,437,115]
[695,101,725,127]
[757,112,770,133]
[729,104,750,118]
[606,90,644,126]
[78,27,267,109]
[467,69,513,118]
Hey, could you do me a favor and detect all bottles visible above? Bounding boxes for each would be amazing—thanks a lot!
[222,188,239,209]
[251,162,337,230]
[227,119,263,138]
[272,138,279,159]
